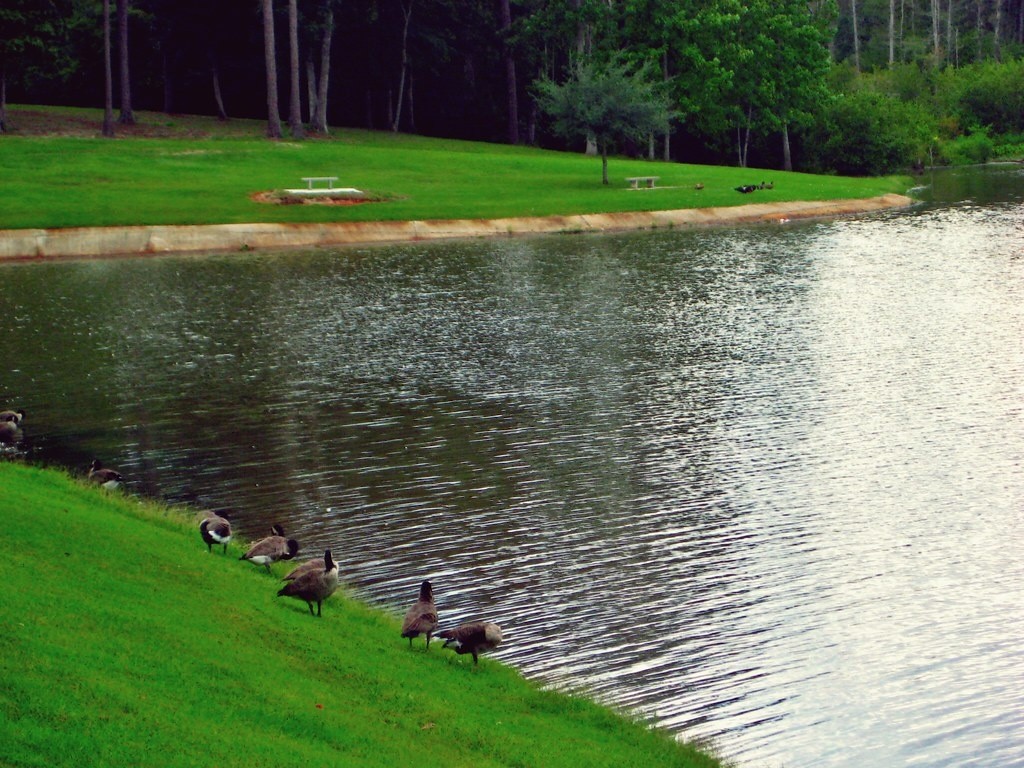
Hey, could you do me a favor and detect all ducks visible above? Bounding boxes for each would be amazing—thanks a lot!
[0,408,25,443]
[199,510,233,554]
[695,183,705,189]
[431,622,504,665]
[88,460,125,490]
[238,523,299,574]
[400,579,439,652]
[733,181,773,194]
[280,556,339,582]
[277,548,340,618]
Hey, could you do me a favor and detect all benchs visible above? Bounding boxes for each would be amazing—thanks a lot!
[626,176,660,188]
[302,177,338,189]
